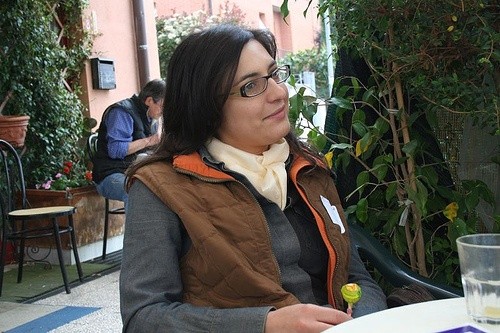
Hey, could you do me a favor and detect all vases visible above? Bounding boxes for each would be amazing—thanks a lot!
[0,115,30,148]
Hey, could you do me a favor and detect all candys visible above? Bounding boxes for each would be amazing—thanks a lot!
[341,283,362,316]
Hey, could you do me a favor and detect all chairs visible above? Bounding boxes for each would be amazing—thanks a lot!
[0,139,85,299]
[87,133,126,260]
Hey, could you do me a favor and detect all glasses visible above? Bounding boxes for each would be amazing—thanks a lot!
[228,65,290,97]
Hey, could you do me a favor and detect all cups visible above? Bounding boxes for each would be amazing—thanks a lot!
[456,233,500,326]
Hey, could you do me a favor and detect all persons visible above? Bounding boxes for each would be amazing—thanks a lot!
[120,25,386,333]
[93,80,167,208]
